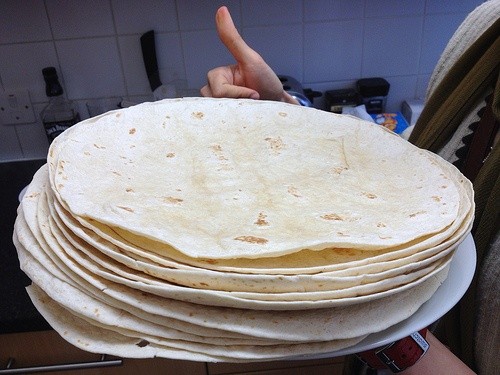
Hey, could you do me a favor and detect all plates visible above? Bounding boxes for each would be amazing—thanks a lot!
[288,231,476,360]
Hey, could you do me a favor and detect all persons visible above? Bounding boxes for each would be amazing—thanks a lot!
[201,0,500,374]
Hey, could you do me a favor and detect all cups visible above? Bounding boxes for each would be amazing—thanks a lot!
[85,96,122,117]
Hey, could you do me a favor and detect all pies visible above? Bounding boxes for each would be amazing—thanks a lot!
[12,96,476,362]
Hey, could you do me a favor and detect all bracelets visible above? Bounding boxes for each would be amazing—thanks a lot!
[353,323,430,375]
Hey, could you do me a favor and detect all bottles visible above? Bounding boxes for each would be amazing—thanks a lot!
[40,65,82,149]
